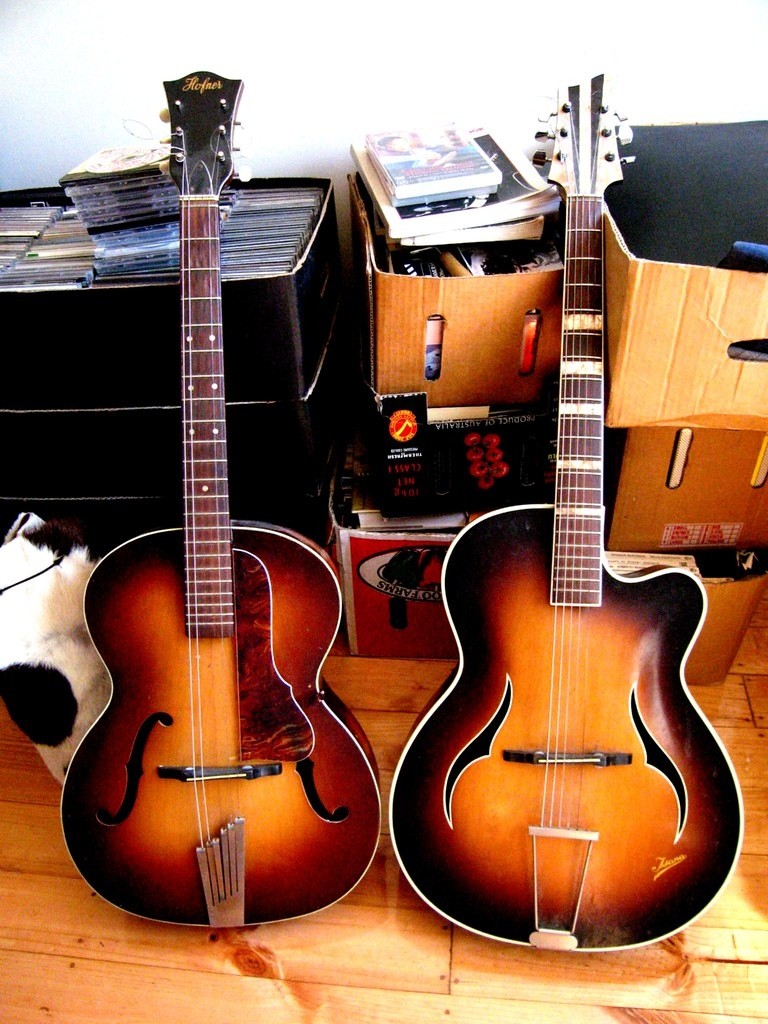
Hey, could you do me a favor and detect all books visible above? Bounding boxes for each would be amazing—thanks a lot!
[350,124,561,246]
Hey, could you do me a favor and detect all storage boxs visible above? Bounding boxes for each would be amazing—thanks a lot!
[604,124,768,687]
[329,171,566,660]
[0,177,343,558]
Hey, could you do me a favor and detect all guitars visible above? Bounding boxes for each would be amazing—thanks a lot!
[386,72,747,956]
[60,68,384,929]
